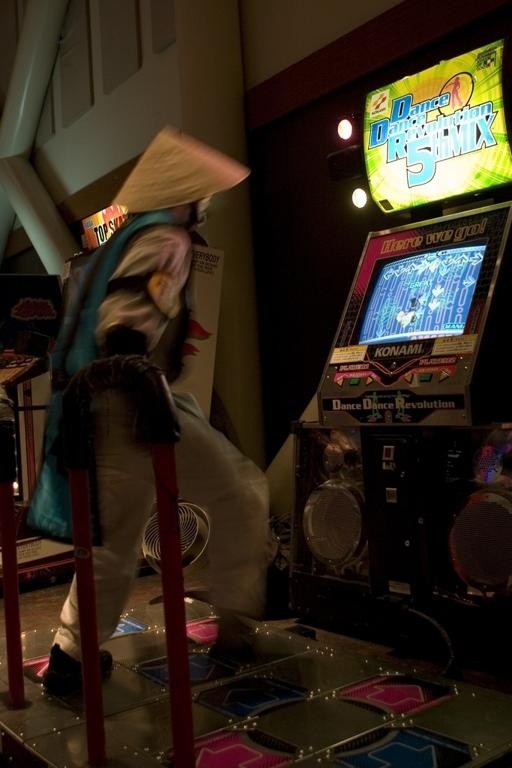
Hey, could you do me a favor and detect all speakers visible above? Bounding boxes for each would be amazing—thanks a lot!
[289,423,399,649]
[402,423,512,680]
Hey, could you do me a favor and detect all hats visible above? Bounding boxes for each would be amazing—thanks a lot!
[115,124,250,212]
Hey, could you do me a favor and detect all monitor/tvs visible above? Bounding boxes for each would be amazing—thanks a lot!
[356,238,489,345]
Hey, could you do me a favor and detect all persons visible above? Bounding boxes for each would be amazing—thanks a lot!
[25,129,270,709]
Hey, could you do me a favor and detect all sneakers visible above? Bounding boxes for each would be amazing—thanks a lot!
[46,642,113,699]
